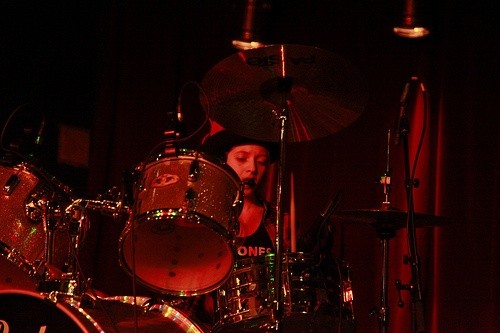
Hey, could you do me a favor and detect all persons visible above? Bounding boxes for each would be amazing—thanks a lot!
[198,133,283,319]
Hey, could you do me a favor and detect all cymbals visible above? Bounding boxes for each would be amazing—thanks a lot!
[197,43,372,142]
[329,207,451,228]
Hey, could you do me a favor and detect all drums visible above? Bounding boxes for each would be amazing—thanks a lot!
[0,157,91,288]
[218,251,352,333]
[0,289,209,333]
[117,149,244,298]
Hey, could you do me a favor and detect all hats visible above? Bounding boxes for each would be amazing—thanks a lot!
[202,129,279,166]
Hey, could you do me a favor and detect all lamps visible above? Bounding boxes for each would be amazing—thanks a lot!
[232,0,270,51]
[394,0,430,38]
